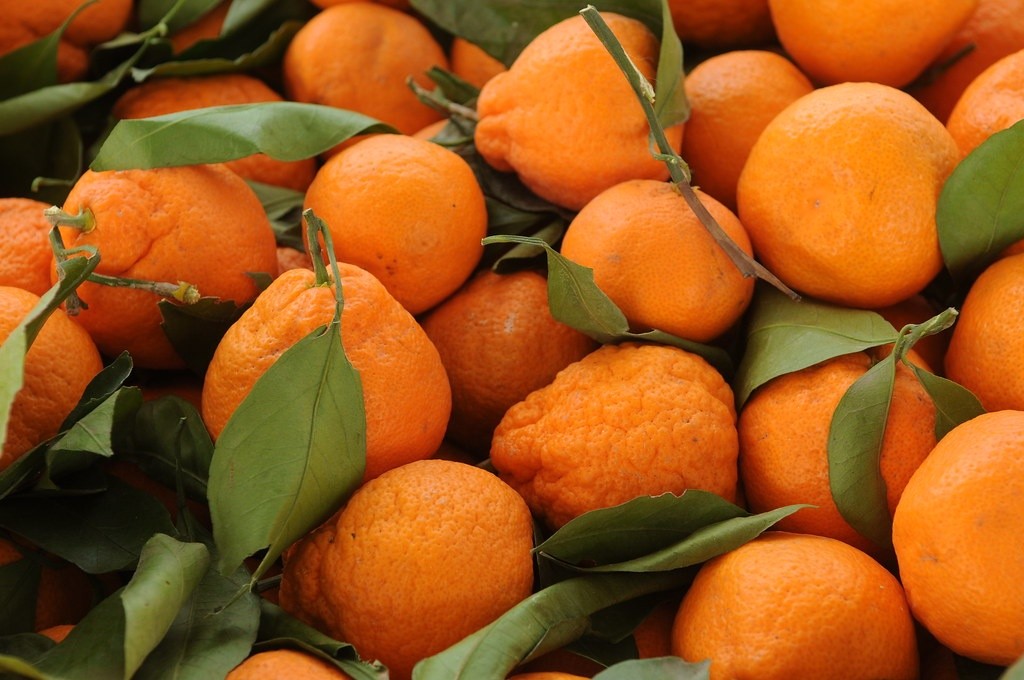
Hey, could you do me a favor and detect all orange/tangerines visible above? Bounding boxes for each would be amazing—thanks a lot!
[0,0,1024,680]
